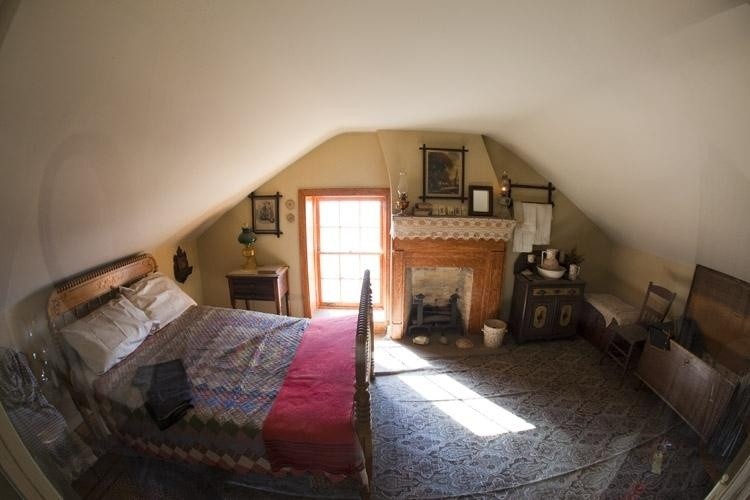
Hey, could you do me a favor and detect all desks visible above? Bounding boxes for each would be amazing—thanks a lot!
[226,265,290,317]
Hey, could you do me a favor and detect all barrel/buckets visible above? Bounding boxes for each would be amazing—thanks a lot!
[481,319,508,349]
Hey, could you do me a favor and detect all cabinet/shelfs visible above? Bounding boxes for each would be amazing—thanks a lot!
[577,292,640,353]
[509,272,585,342]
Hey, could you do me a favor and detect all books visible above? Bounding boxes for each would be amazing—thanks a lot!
[415,202,433,208]
[412,207,432,216]
[257,262,286,274]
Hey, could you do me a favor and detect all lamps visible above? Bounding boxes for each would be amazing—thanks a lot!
[497,170,511,217]
[396,172,411,216]
[238,227,258,268]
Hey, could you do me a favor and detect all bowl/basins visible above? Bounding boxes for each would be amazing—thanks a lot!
[536,264,566,279]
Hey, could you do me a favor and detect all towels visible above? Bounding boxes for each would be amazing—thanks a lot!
[513,201,552,253]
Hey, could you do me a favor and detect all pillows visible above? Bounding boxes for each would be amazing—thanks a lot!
[59,292,153,374]
[110,271,197,336]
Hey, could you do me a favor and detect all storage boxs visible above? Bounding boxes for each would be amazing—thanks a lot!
[635,263,750,445]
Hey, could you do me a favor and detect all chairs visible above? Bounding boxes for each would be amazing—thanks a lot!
[597,281,676,389]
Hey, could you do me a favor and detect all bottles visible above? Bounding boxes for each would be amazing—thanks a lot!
[568,264,577,281]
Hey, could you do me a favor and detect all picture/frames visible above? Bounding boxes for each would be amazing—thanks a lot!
[248,192,283,238]
[418,143,469,204]
[469,185,494,216]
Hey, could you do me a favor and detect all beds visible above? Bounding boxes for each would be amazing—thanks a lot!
[47,253,374,500]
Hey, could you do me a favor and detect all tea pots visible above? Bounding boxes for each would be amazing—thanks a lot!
[540,245,561,272]
